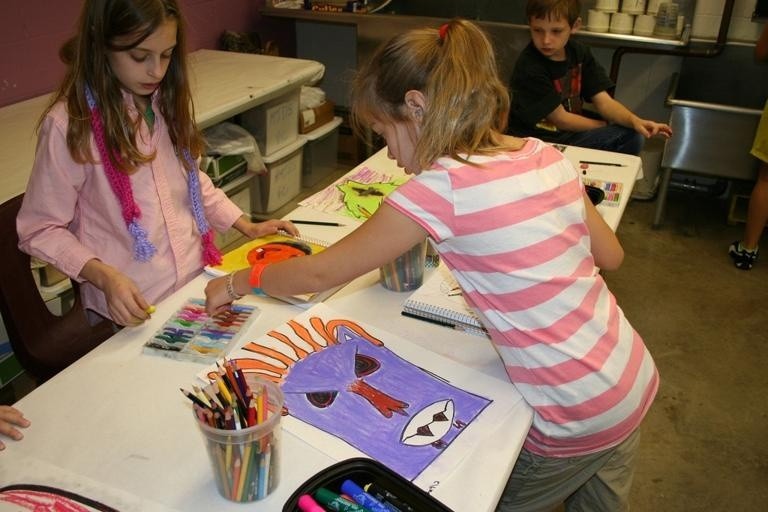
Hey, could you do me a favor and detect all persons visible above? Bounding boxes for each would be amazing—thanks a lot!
[15,1,300,330]
[203,18,660,512]
[0,404,30,451]
[504,0,673,157]
[726,22,768,271]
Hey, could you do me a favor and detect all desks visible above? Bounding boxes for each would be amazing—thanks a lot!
[0,141,645,512]
[0,49,325,296]
[259,0,685,202]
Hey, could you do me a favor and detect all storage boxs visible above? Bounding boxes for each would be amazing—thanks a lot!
[211,174,258,252]
[259,137,308,214]
[302,116,343,188]
[234,84,300,159]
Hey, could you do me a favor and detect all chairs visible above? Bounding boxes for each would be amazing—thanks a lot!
[0,194,127,385]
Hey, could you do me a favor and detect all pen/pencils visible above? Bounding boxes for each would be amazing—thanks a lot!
[297,477,415,512]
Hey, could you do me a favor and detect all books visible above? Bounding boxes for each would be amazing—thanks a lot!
[399,264,489,340]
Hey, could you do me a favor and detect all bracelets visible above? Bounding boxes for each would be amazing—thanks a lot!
[224,269,245,301]
[249,263,266,299]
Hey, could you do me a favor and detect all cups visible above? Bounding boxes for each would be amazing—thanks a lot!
[374,236,425,292]
[192,371,285,505]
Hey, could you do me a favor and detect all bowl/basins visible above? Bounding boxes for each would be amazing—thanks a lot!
[588,0,672,38]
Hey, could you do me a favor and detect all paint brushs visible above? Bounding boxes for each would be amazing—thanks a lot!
[579,160,625,168]
[401,309,467,332]
[179,355,277,503]
[288,219,346,227]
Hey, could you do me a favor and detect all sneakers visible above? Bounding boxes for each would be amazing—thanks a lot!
[729,240,759,271]
